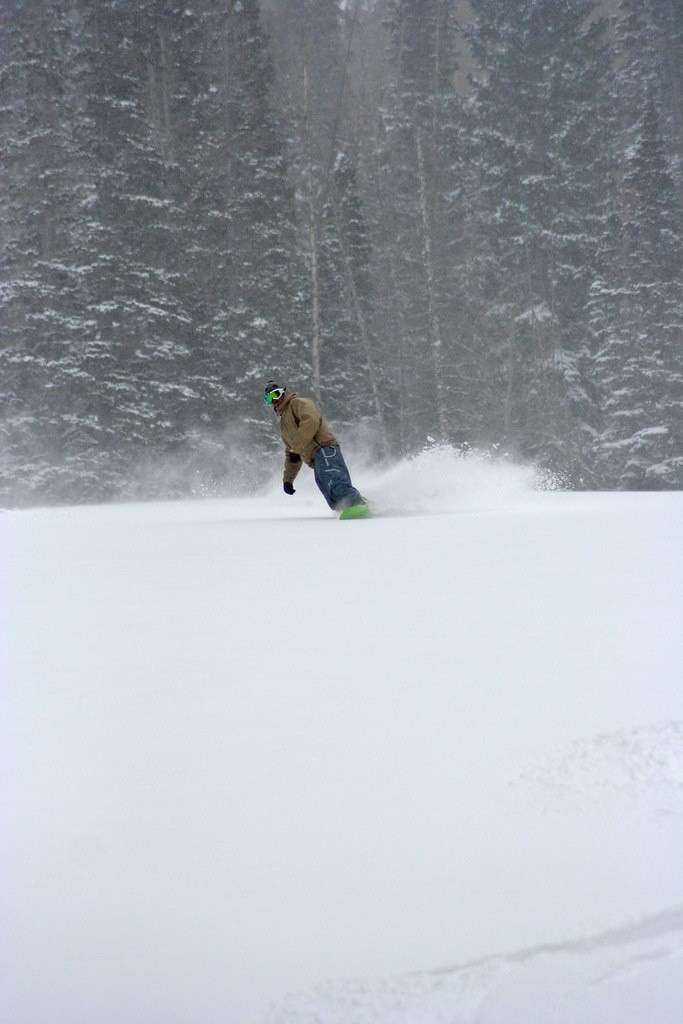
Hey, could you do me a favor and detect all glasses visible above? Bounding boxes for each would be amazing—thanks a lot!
[264,388,286,404]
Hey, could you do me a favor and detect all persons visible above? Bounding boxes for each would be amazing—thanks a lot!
[265,380,365,509]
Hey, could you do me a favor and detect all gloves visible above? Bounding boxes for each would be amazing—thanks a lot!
[289,450,301,464]
[284,482,296,495]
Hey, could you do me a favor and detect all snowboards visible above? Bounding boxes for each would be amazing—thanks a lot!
[340,503,371,521]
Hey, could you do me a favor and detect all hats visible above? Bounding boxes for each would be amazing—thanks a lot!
[265,380,284,395]
